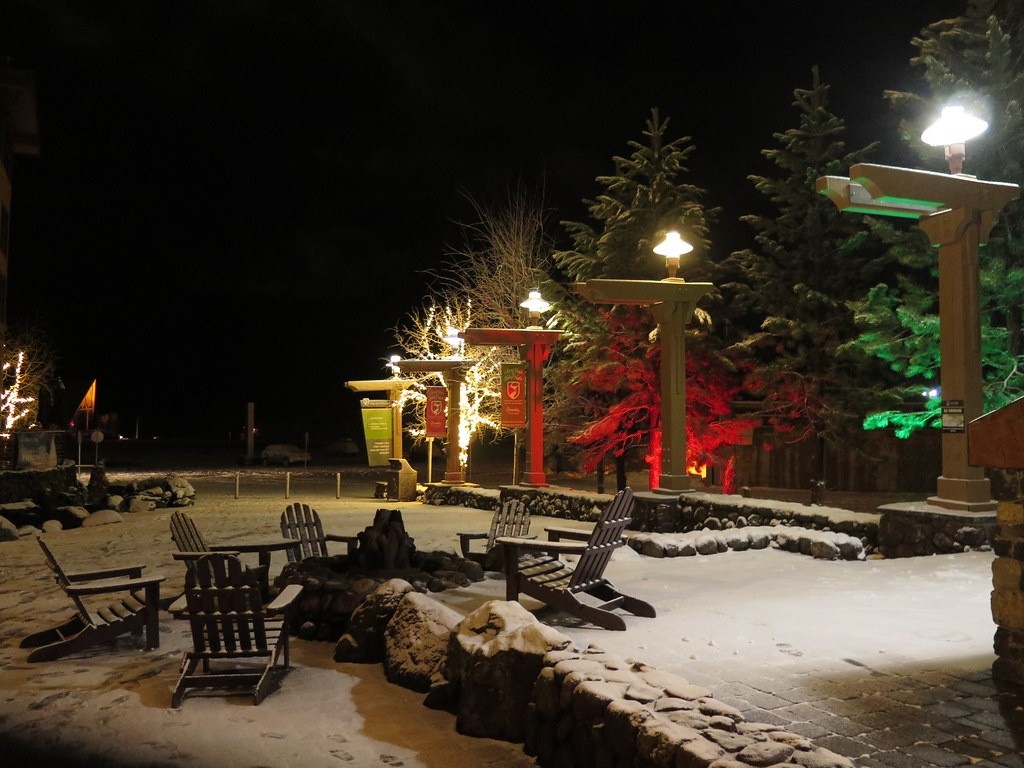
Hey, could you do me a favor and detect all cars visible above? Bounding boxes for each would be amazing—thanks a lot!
[261,444,312,468]
[323,438,360,458]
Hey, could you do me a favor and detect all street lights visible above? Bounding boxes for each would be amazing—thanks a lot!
[345,355,414,460]
[814,106,1020,511]
[392,328,481,484]
[570,230,714,495]
[457,292,565,488]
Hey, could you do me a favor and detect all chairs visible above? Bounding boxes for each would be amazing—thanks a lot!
[170,510,241,579]
[19,534,167,663]
[495,486,657,631]
[456,500,537,563]
[170,553,303,710]
[280,503,358,562]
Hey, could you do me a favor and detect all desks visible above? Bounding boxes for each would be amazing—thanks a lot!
[206,539,302,604]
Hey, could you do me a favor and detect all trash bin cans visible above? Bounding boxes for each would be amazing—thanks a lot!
[384,457,418,502]
